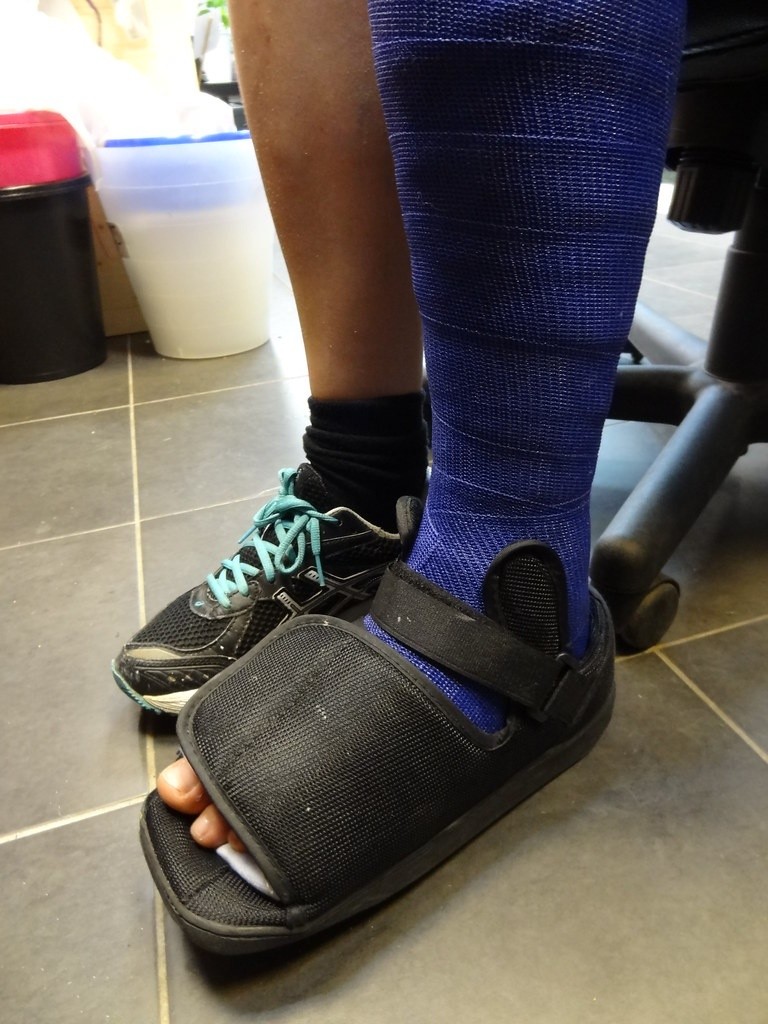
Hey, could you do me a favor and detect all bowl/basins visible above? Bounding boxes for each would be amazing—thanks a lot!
[0,110,87,185]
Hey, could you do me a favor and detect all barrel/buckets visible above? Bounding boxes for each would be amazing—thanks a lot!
[0,167,107,386]
[91,138,273,360]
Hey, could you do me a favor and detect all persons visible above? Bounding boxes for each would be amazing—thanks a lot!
[112,0,767,955]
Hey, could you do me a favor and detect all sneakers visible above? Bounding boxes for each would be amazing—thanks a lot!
[112,463,430,716]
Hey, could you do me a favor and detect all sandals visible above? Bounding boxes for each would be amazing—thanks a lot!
[139,495,616,962]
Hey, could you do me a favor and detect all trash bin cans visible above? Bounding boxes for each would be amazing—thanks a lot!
[0,170,110,386]
[86,132,274,360]
